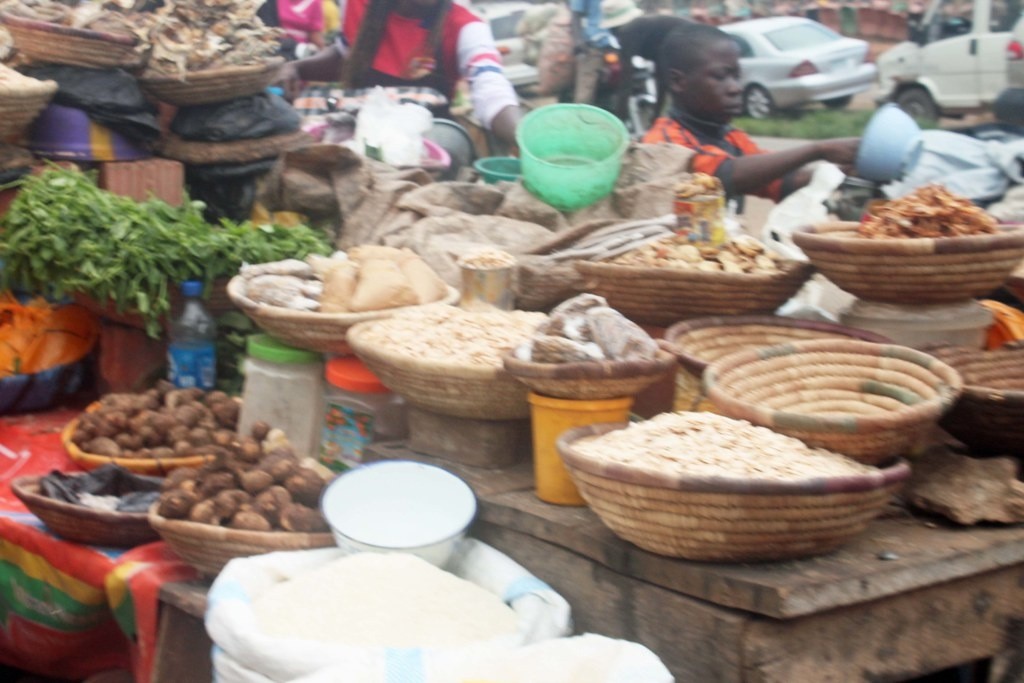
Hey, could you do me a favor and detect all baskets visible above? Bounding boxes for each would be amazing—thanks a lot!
[500,337,676,399]
[62,395,222,478]
[554,422,912,564]
[701,338,963,466]
[227,243,460,353]
[920,344,1024,455]
[344,318,531,421]
[663,316,898,407]
[790,219,1024,307]
[0,10,143,71]
[12,470,156,549]
[146,498,339,576]
[570,231,816,326]
[0,63,58,146]
[138,55,285,105]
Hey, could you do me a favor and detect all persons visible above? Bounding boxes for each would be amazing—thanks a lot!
[276,0,527,193]
[640,25,862,232]
[564,1,687,129]
[253,0,339,63]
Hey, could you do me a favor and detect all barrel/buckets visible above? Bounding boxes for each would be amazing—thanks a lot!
[526,391,635,505]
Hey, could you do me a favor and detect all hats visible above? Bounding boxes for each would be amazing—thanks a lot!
[597,0,644,30]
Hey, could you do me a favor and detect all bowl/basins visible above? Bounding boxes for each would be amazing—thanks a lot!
[475,155,520,182]
[321,461,477,570]
[516,105,629,211]
[15,101,154,160]
[854,102,923,182]
[423,117,476,168]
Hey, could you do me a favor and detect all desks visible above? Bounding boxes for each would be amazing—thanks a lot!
[0,404,213,683]
[369,436,1024,683]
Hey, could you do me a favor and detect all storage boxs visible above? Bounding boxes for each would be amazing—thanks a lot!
[99,157,187,210]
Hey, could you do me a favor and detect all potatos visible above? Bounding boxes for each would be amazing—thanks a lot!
[71,378,327,533]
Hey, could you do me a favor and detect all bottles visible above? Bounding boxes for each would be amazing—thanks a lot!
[218,310,249,390]
[236,333,324,456]
[167,280,215,390]
[317,354,407,476]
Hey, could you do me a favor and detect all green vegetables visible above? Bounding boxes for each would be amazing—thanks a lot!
[0,159,333,336]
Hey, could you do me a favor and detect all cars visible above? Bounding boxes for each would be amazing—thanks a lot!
[873,0,1024,131]
[711,17,877,123]
[464,3,545,87]
